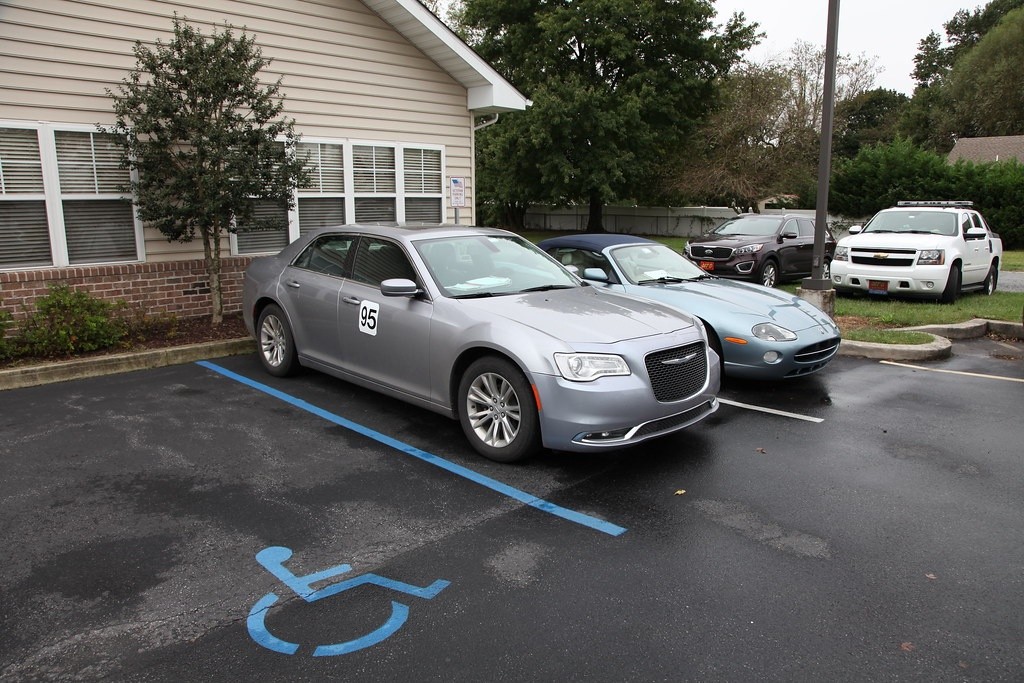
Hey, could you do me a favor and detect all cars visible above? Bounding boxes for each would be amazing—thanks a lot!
[683,213,839,292]
[241,222,720,464]
[534,232,840,387]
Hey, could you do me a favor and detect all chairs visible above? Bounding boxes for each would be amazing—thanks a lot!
[378,245,421,287]
[430,243,475,287]
[561,252,586,278]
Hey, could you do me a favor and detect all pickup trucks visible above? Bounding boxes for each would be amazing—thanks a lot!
[829,201,1001,305]
